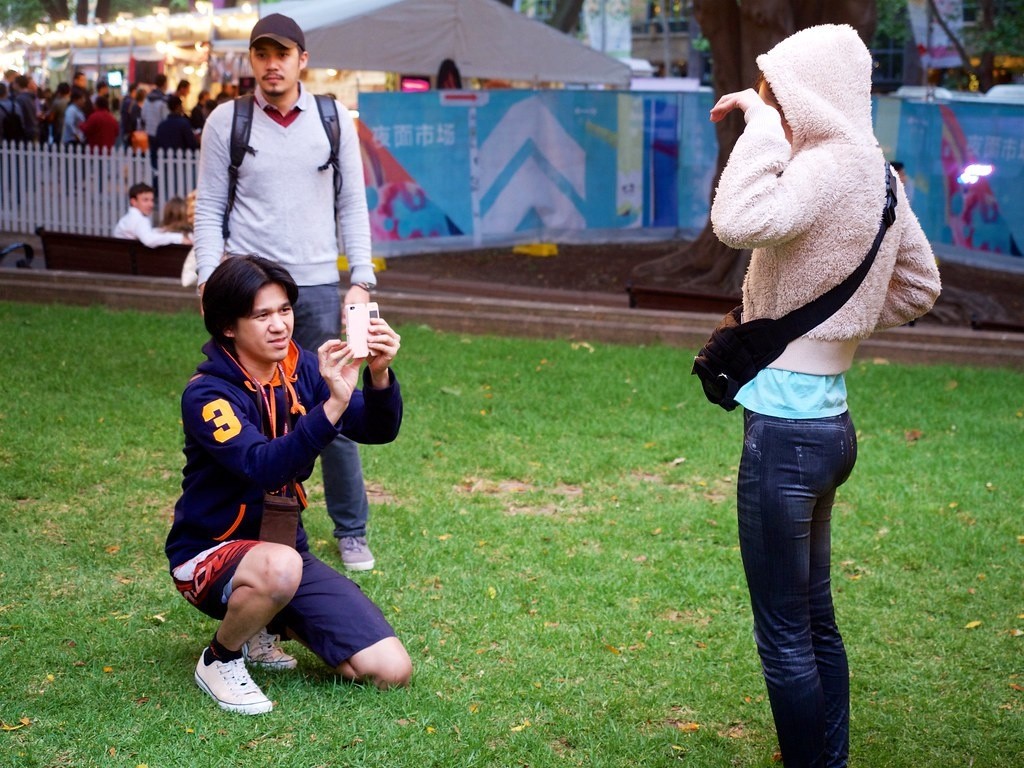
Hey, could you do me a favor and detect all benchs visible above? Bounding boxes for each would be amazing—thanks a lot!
[623,284,743,316]
[32,226,193,279]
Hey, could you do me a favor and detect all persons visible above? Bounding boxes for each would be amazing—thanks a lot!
[110,181,196,249]
[164,252,415,716]
[190,14,381,573]
[0,67,255,217]
[691,23,943,768]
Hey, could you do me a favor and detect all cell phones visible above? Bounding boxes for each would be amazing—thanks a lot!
[345,303,370,359]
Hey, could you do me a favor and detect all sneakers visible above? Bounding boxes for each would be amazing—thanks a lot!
[194,646,272,715]
[337,532,376,571]
[241,628,296,673]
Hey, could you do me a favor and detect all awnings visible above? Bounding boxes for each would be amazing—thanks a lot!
[258,0,636,90]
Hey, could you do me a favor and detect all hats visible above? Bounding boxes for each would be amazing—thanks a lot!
[249,13,309,53]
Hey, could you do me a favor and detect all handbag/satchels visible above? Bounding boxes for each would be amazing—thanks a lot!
[693,305,783,410]
